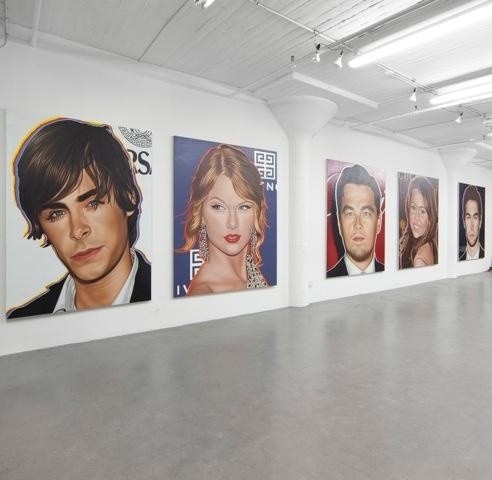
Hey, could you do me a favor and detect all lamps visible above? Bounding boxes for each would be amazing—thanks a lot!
[313,0,492,125]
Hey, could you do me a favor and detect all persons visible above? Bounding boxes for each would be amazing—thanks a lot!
[459,185,485,261]
[174,143,271,296]
[399,175,438,269]
[327,164,385,278]
[6,117,151,319]
